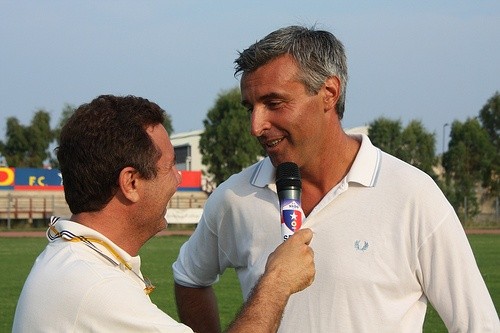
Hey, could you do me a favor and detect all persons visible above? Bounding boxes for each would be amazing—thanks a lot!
[170,26,500,332]
[10,94,316,332]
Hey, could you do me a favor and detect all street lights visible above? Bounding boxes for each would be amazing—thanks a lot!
[442,122,449,154]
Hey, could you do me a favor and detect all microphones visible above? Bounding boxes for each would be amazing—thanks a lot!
[275,162,302,243]
